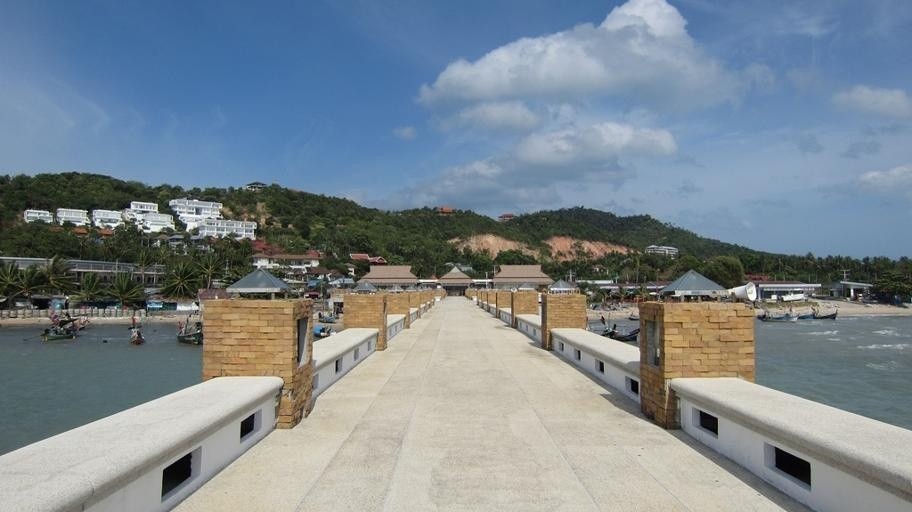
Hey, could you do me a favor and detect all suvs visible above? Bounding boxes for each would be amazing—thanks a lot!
[327,277,356,288]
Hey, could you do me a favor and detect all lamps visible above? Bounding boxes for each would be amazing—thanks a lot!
[389,284,404,293]
[551,279,575,294]
[355,280,378,293]
[518,282,534,292]
[226,266,290,300]
[660,267,729,302]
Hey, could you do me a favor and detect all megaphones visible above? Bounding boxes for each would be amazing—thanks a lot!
[728,280,758,303]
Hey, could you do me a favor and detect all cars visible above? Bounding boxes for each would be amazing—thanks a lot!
[303,288,331,300]
[350,289,377,295]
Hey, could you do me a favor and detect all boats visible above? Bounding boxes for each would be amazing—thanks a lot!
[39,302,338,346]
[601,325,641,342]
[628,314,640,321]
[756,307,841,322]
